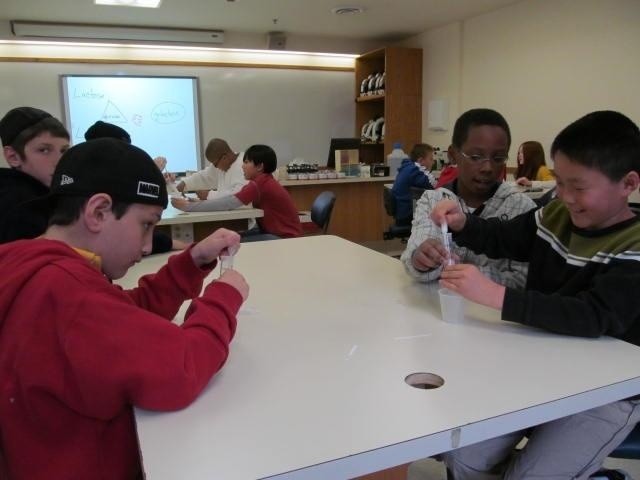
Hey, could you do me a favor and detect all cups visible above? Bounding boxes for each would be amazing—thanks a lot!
[289,169,346,180]
[437,288,465,324]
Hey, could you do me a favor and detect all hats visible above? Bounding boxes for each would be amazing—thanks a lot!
[83,120,130,140]
[0,107,51,144]
[15,138,169,212]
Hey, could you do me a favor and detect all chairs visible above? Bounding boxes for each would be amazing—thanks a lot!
[384,183,411,240]
[301,190,337,236]
[409,186,427,215]
[525,424,640,459]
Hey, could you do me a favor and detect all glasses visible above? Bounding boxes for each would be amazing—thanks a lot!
[455,148,510,167]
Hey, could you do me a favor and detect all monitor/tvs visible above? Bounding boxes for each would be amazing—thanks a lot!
[327,138,361,168]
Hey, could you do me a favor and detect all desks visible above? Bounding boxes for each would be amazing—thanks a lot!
[111,235,640,480]
[155,191,264,249]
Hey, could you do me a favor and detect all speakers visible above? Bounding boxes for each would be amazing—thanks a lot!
[267,32,286,50]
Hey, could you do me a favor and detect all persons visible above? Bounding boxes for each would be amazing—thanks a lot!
[1,137,253,480]
[175,137,245,200]
[0,106,72,248]
[390,142,440,227]
[514,139,557,191]
[400,106,539,292]
[169,143,306,242]
[428,109,640,479]
[431,144,507,193]
[83,118,175,188]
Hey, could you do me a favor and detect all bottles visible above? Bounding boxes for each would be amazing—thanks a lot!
[219,255,236,278]
[387,143,409,178]
[440,233,453,270]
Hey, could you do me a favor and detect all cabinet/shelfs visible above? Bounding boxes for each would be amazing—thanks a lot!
[355,48,422,165]
